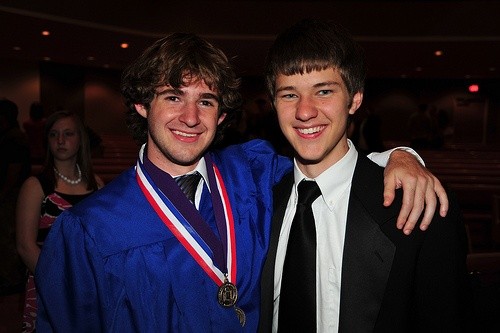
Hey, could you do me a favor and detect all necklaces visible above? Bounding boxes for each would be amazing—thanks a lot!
[53,161,83,184]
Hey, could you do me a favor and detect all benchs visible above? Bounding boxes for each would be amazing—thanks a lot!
[383,139,500,287]
[89,134,140,185]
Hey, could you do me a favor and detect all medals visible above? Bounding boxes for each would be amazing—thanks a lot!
[235,306,247,329]
[218,281,238,308]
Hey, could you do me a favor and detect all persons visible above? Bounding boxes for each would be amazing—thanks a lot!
[254,27,469,332]
[15,108,106,333]
[34,31,449,333]
[0,98,119,298]
[220,84,469,158]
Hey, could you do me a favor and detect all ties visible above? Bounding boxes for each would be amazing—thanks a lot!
[276,179,322,333]
